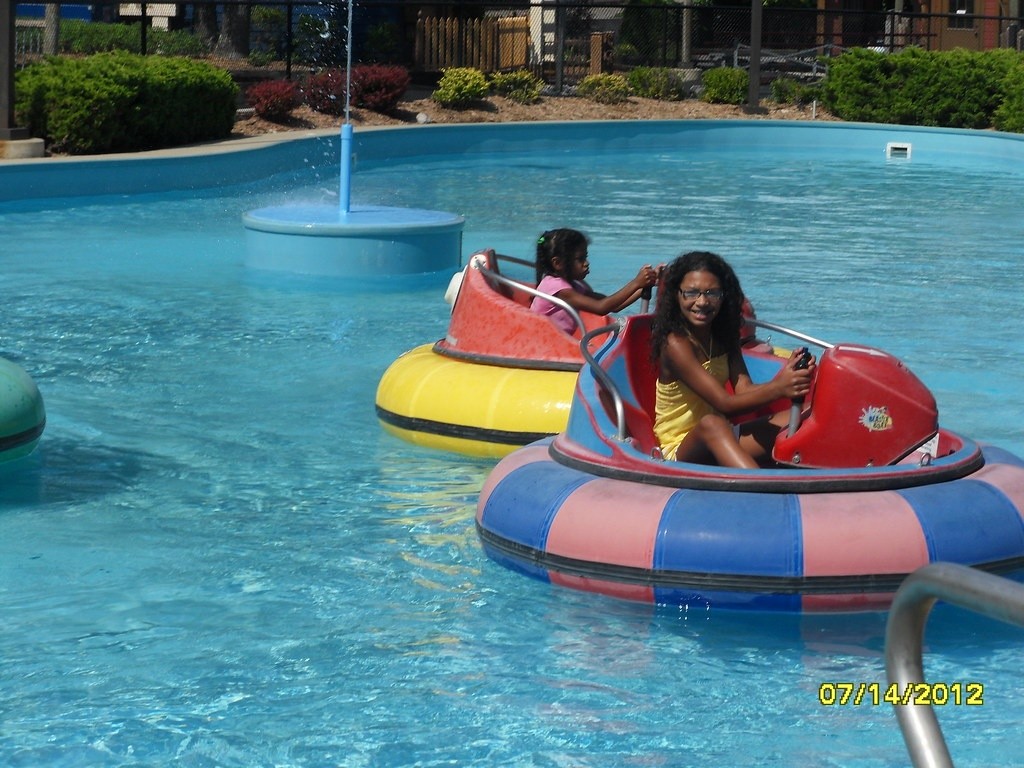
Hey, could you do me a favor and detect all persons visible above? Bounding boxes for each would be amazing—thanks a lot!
[528,228,668,337]
[647,251,817,468]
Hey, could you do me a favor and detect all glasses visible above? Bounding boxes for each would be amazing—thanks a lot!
[677,287,724,299]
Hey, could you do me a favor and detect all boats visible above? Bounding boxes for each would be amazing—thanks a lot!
[474,269,1023,612]
[0,355,46,461]
[375,248,666,459]
